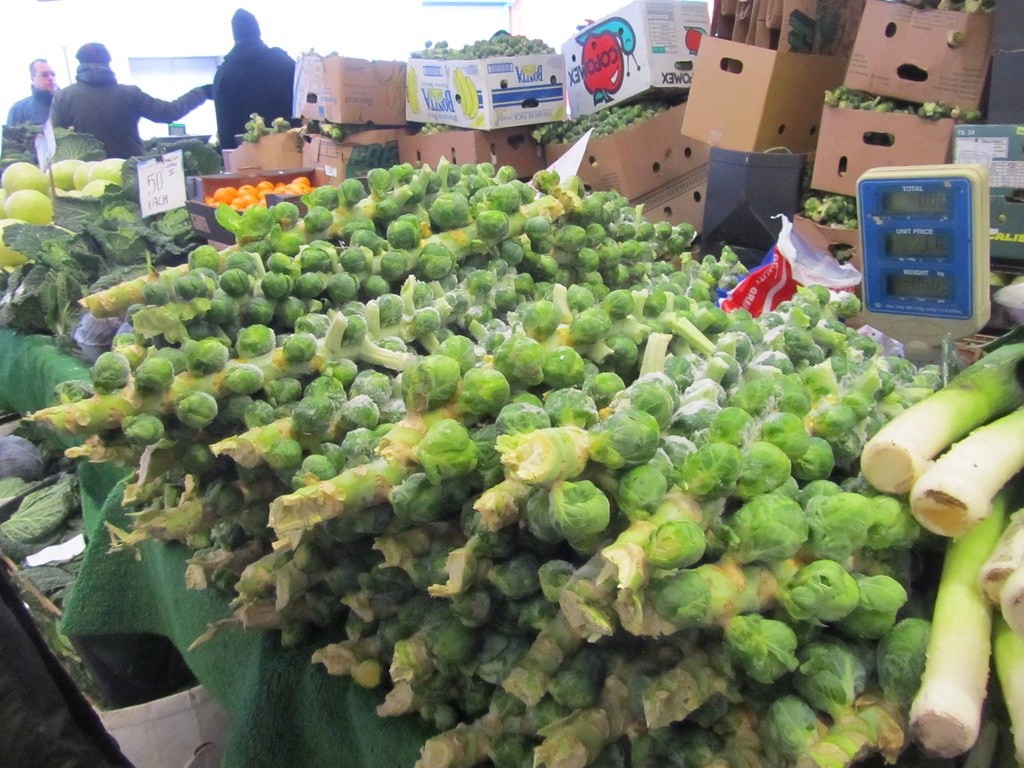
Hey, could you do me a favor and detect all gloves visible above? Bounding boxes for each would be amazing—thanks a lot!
[201,84,215,99]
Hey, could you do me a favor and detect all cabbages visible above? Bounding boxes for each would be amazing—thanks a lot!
[802,84,981,230]
[418,32,673,142]
[56,203,949,768]
[0,182,200,348]
[0,432,45,483]
[0,473,86,612]
[302,162,624,203]
[1,122,223,176]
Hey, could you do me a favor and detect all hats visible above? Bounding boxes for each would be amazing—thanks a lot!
[232,7,261,40]
[74,42,112,64]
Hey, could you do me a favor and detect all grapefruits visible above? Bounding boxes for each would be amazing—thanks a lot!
[0,157,135,267]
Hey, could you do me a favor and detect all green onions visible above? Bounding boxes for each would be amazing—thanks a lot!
[862,341,1024,766]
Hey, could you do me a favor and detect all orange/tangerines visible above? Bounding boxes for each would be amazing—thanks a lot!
[204,176,318,210]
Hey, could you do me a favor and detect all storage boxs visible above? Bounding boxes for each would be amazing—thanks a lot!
[258,133,303,169]
[989,196,1024,259]
[951,122,1024,199]
[184,200,263,247]
[843,1,992,111]
[402,55,566,128]
[791,211,858,296]
[564,0,710,113]
[290,54,406,126]
[678,35,847,155]
[394,130,545,183]
[698,145,805,266]
[624,165,708,251]
[188,168,314,199]
[303,129,410,185]
[540,101,711,202]
[809,105,954,198]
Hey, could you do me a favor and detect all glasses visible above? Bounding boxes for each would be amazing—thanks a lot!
[33,72,56,79]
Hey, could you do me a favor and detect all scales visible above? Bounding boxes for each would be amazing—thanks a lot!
[854,162,989,370]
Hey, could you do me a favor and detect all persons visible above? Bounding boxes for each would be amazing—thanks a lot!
[213,8,303,150]
[51,42,213,159]
[7,59,61,126]
